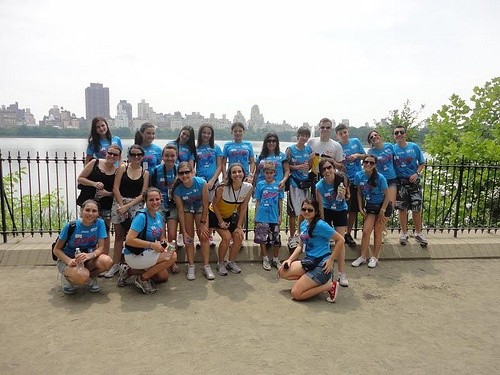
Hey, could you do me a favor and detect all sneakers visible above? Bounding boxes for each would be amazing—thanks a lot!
[177,233,216,247]
[368,257,378,268]
[352,257,367,266]
[401,235,409,245]
[261,256,271,270]
[336,273,349,286]
[271,256,282,269]
[326,281,339,302]
[288,235,300,248]
[413,232,428,246]
[61,260,241,295]
[345,233,357,247]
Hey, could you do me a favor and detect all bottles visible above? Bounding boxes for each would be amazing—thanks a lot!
[75,247,84,270]
[336,182,344,201]
[376,154,383,168]
[303,160,309,177]
[116,204,125,222]
[163,240,176,260]
[408,175,417,185]
[94,188,100,201]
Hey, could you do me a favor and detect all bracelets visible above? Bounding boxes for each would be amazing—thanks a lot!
[200,220,206,223]
[149,242,152,248]
[93,252,97,258]
[248,173,254,177]
[415,172,420,176]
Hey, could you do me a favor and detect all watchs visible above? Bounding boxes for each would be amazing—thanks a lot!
[236,225,242,229]
[381,208,386,212]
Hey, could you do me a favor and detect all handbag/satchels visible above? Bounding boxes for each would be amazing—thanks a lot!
[124,212,147,257]
[51,219,77,261]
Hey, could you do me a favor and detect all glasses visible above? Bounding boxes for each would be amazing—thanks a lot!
[267,139,278,144]
[394,131,406,135]
[178,170,191,175]
[231,170,244,174]
[129,153,143,157]
[302,208,316,213]
[108,152,118,157]
[363,160,376,165]
[370,134,378,139]
[322,164,332,172]
[320,126,332,130]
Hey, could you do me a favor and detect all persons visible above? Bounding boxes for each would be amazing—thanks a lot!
[277,197,345,302]
[86,116,123,173]
[315,159,349,287]
[129,122,163,173]
[173,118,397,276]
[392,126,428,246]
[149,144,181,272]
[53,199,113,294]
[77,145,122,277]
[117,187,177,294]
[174,161,216,280]
[351,155,390,267]
[105,145,150,277]
